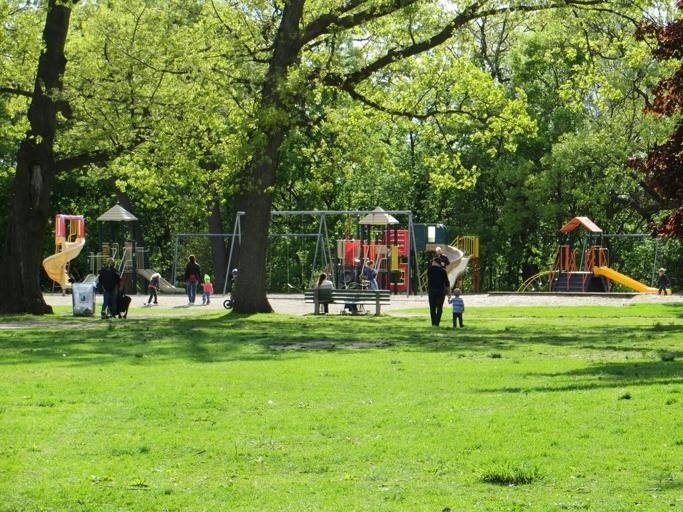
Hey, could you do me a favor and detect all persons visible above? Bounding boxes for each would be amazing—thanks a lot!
[362,259,379,290]
[315,271,334,315]
[200,274,212,305]
[448,289,467,327]
[231,268,238,289]
[657,267,669,294]
[426,257,449,325]
[99,258,120,319]
[436,247,450,268]
[183,255,201,305]
[148,273,160,306]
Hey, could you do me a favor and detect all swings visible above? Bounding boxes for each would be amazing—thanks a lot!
[359,213,389,286]
[285,214,306,287]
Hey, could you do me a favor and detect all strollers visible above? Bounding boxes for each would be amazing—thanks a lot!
[108,275,131,319]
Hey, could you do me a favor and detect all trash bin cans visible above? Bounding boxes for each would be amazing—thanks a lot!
[72,282,97,315]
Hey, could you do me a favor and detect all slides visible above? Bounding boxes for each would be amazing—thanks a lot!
[593,266,671,295]
[42,237,85,288]
[136,269,186,293]
[442,244,473,292]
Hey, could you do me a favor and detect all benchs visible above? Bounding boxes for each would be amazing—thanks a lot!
[301,286,392,318]
